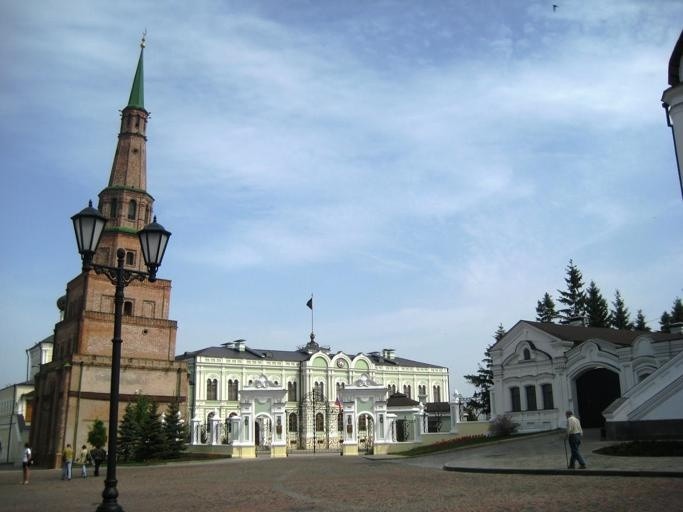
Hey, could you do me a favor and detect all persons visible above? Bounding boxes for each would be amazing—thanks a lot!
[90,443,104,476]
[60,443,73,480]
[563,410,587,469]
[22,442,31,484]
[77,444,91,481]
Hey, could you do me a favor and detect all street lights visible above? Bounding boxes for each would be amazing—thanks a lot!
[68,199,171,512]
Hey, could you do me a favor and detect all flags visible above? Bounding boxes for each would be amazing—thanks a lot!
[334,398,342,413]
[305,298,311,310]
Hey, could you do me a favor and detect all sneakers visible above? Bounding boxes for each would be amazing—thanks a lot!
[568,464,586,469]
